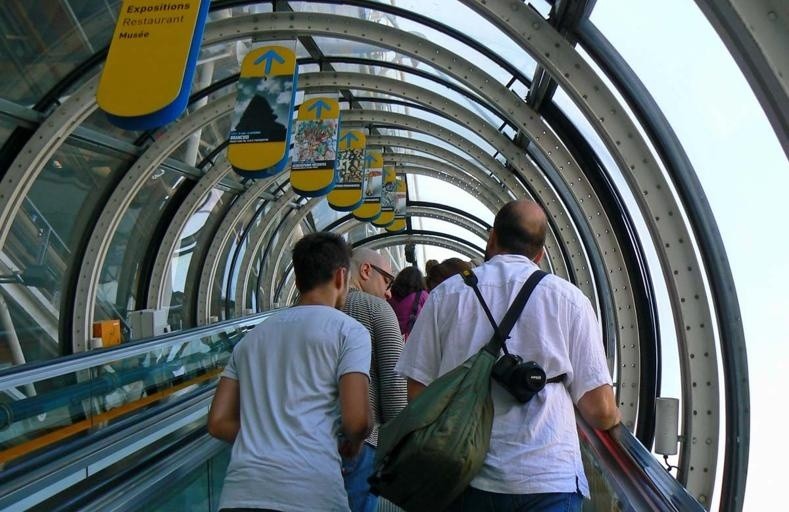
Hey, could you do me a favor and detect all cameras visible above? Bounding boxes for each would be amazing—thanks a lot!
[490,353,547,404]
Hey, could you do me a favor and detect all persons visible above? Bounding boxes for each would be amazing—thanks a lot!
[423,259,439,283]
[386,267,430,344]
[429,258,469,291]
[339,246,409,511]
[392,196,624,512]
[204,231,372,510]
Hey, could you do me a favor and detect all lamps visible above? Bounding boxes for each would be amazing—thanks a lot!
[653,394,685,476]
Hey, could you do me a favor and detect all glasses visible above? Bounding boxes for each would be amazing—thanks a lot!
[369,263,395,290]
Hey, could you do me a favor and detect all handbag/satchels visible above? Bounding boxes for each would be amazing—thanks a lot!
[367,349,498,512]
